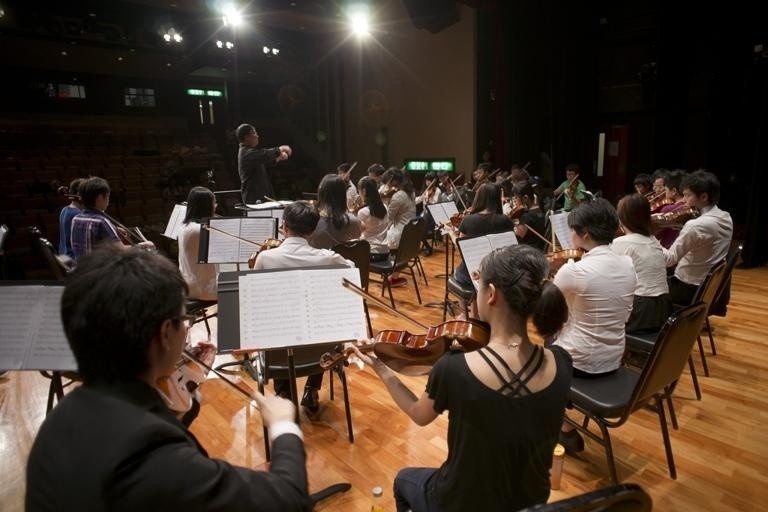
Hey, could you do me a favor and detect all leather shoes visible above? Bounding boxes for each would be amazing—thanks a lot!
[302,388,319,412]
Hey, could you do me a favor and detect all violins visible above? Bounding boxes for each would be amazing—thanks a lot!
[144,331,215,420]
[472,247,587,281]
[619,206,701,233]
[319,320,490,371]
[379,186,400,198]
[248,239,280,269]
[422,187,435,204]
[434,207,472,231]
[645,192,674,213]
[566,180,579,201]
[506,205,529,222]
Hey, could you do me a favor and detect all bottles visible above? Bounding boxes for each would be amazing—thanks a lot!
[552,444,565,490]
[370,487,386,511]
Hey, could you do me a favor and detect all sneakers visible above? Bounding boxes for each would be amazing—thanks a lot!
[558,430,584,452]
[388,277,407,288]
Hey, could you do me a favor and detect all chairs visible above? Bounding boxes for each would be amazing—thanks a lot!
[256,346,355,462]
[29,226,71,281]
[522,483,654,511]
[335,215,428,314]
[441,275,478,323]
[568,242,744,486]
[186,298,223,334]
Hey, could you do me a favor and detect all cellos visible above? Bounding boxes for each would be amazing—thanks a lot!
[50,178,146,247]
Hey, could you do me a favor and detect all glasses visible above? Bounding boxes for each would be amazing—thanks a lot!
[652,185,664,191]
[154,315,196,329]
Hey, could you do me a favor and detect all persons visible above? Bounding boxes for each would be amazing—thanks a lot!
[235,123,293,203]
